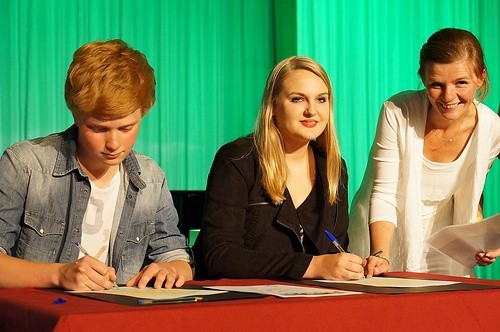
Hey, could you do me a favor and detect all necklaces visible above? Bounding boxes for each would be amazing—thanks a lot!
[427,101,470,142]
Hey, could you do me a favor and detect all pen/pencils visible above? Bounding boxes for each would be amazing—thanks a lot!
[76,243,119,289]
[326,231,365,278]
[138,297,203,305]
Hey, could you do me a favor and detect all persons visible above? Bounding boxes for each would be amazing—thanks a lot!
[346,27,500,280]
[194,55,367,281]
[0,39,197,291]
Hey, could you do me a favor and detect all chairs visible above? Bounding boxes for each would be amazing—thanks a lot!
[139,190,207,272]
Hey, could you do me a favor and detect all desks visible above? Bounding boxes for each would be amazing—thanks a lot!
[0,272,500,332]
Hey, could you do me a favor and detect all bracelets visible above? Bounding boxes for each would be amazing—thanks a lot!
[371,251,392,266]
